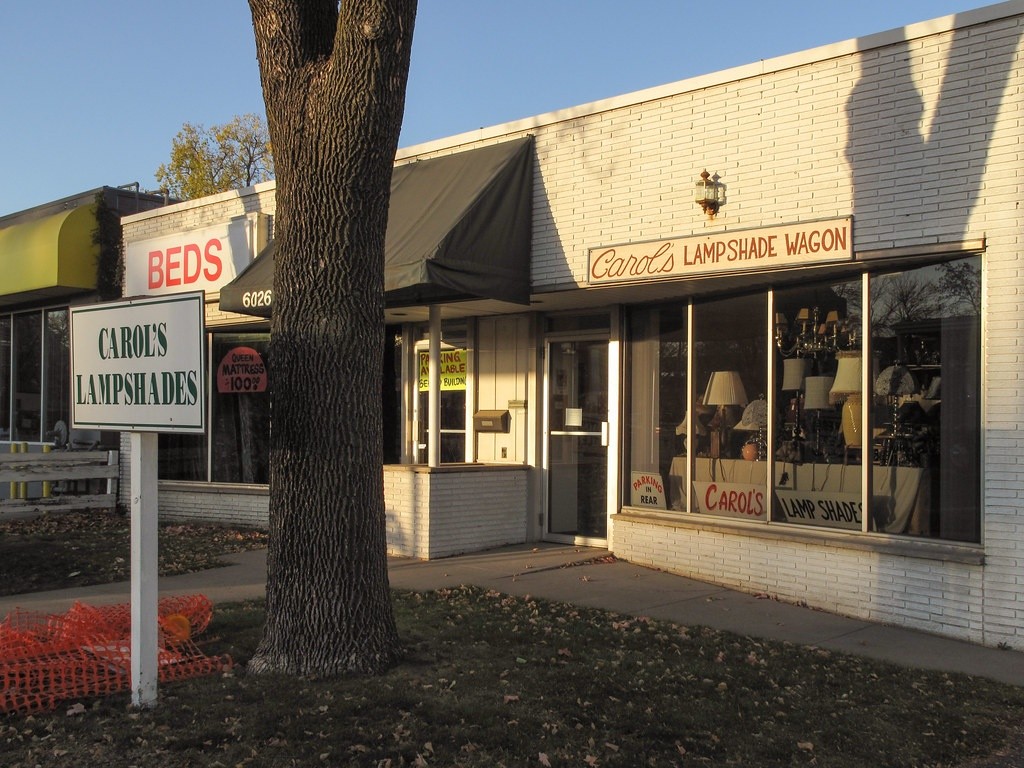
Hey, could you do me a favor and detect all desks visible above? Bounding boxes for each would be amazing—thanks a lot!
[670,456,934,539]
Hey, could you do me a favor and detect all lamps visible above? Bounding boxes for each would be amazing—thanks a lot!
[701,308,942,465]
[695,168,718,216]
